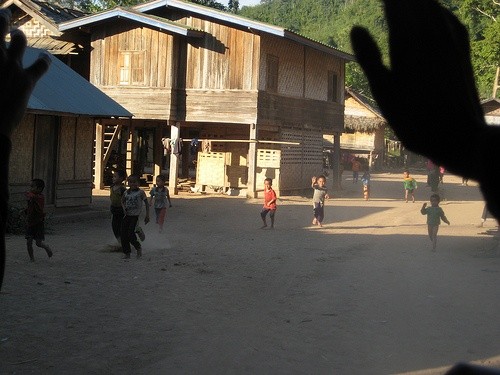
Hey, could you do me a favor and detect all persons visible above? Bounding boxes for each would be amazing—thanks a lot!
[120,173,150,259]
[477,202,498,229]
[0,6,51,298]
[149,175,172,231]
[402,168,418,203]
[24,179,53,262]
[426,160,469,192]
[349,0,500,375]
[311,175,329,227]
[351,157,361,184]
[360,168,370,200]
[420,194,450,251]
[109,168,145,243]
[260,177,277,229]
